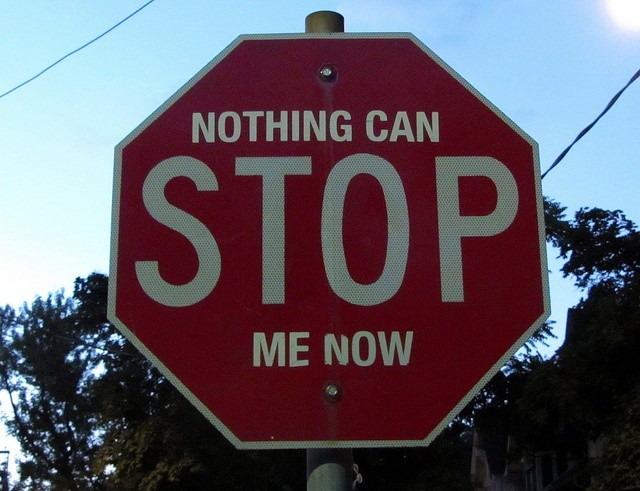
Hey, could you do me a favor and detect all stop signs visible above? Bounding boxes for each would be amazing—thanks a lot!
[106,33,551,450]
